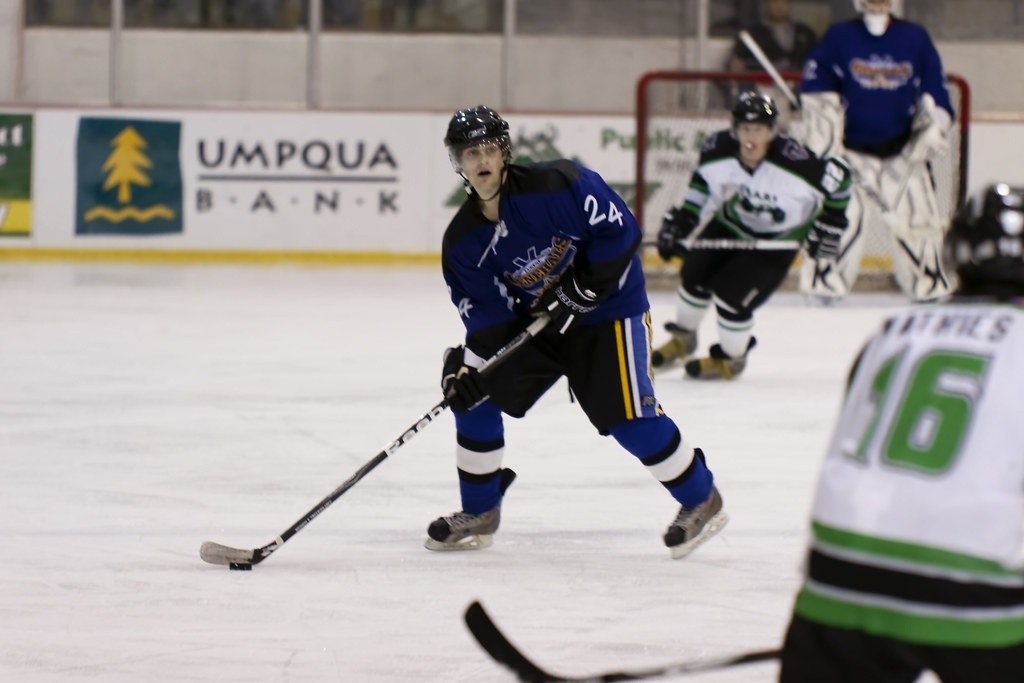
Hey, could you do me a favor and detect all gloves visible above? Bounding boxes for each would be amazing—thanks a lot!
[441,346,480,410]
[804,213,848,258]
[657,207,693,261]
[541,265,606,341]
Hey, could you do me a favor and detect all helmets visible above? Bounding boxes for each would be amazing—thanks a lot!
[443,105,513,154]
[853,0,893,14]
[940,184,1024,275]
[732,91,778,122]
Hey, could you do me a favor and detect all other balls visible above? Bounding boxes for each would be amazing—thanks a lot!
[229,562,252,571]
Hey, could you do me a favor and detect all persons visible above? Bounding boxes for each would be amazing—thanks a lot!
[791,0,961,305]
[714,0,817,112]
[651,94,853,382]
[776,176,1024,683]
[426,101,722,547]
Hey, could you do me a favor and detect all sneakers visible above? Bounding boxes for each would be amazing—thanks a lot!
[684,337,757,383]
[425,468,516,551]
[652,323,697,370]
[664,447,730,560]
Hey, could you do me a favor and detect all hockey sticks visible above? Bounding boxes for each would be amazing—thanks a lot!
[199,300,560,564]
[739,29,919,221]
[465,602,784,683]
[641,238,807,250]
